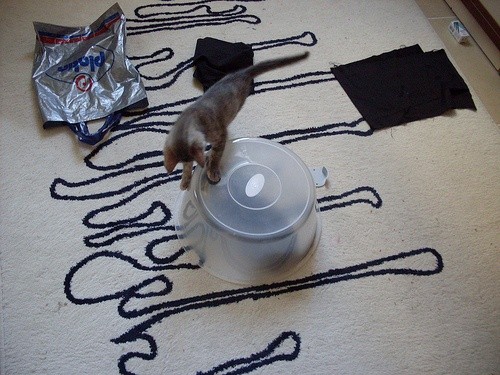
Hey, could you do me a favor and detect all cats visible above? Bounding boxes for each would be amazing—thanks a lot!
[164,51,311,189]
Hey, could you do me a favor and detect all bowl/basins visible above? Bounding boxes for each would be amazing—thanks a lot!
[174,138,321,287]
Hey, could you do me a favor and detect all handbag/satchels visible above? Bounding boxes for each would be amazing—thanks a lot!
[32,2,150,144]
